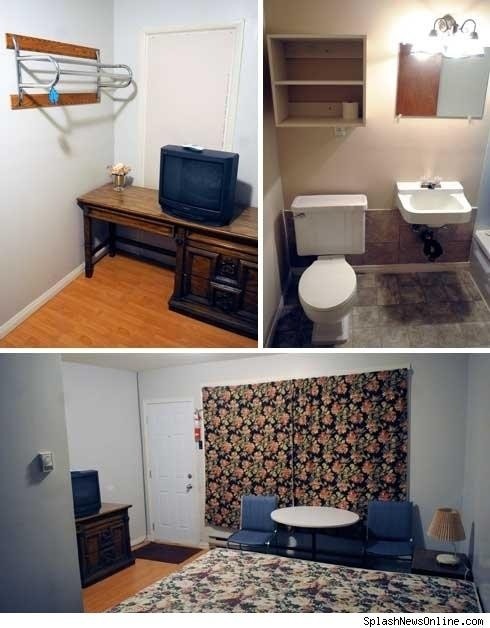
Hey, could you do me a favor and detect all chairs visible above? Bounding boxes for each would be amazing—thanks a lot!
[363,499,415,572]
[227,495,278,552]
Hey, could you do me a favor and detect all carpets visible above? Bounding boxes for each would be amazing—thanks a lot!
[133,539,204,564]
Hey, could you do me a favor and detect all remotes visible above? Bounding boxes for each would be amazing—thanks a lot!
[184,147,201,152]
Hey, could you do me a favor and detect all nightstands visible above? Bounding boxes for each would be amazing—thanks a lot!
[413,549,473,578]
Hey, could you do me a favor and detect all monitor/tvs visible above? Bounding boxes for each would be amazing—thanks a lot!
[159,145,239,226]
[71,470,101,518]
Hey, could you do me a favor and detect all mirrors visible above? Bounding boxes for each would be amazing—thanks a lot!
[437,45,490,119]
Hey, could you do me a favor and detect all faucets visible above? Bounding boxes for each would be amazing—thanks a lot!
[419,173,445,191]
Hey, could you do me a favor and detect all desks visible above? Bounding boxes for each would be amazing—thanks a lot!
[270,505,361,560]
[77,181,258,340]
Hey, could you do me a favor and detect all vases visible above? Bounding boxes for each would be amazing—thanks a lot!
[112,176,127,192]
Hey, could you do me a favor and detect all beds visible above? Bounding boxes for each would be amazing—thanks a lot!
[98,545,485,613]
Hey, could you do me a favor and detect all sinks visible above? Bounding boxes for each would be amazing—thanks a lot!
[397,180,472,227]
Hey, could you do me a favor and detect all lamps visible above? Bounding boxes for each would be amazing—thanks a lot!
[428,505,466,566]
[409,13,485,61]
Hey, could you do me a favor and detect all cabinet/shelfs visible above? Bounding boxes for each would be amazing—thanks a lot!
[267,32,368,126]
[75,501,135,588]
[395,42,444,117]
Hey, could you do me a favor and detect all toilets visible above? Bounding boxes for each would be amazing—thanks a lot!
[291,192,368,342]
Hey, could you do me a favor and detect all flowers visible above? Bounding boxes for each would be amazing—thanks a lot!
[109,163,132,176]
